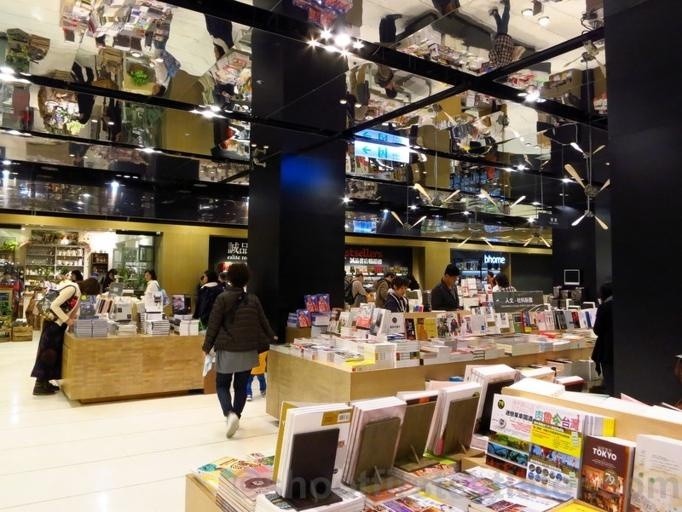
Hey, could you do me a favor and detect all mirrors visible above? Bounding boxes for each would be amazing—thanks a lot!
[3,0,607,243]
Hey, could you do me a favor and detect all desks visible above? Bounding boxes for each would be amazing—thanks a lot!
[265,329,605,436]
[184,450,680,512]
[63,326,218,404]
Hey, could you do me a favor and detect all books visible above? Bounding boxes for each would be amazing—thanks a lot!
[192,358,681,512]
[270,277,598,370]
[74,289,202,339]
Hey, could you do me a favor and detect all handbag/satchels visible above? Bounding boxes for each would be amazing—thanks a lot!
[35,285,77,322]
[155,283,168,305]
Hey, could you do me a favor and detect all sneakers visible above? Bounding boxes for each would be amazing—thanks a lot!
[225,412,240,438]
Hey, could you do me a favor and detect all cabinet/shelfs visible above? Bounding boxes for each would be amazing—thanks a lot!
[1,242,109,342]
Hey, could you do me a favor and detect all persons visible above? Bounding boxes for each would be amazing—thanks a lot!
[432,262,463,311]
[70,60,96,124]
[70,270,83,281]
[376,272,419,312]
[100,268,117,293]
[68,143,89,167]
[372,14,403,98]
[479,0,514,83]
[487,272,510,290]
[101,98,121,141]
[593,282,615,395]
[345,274,368,306]
[141,271,160,301]
[192,262,269,439]
[31,276,100,395]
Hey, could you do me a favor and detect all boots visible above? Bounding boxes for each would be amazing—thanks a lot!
[48,382,59,390]
[33,382,54,394]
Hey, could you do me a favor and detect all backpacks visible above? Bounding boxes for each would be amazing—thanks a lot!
[344,280,359,305]
